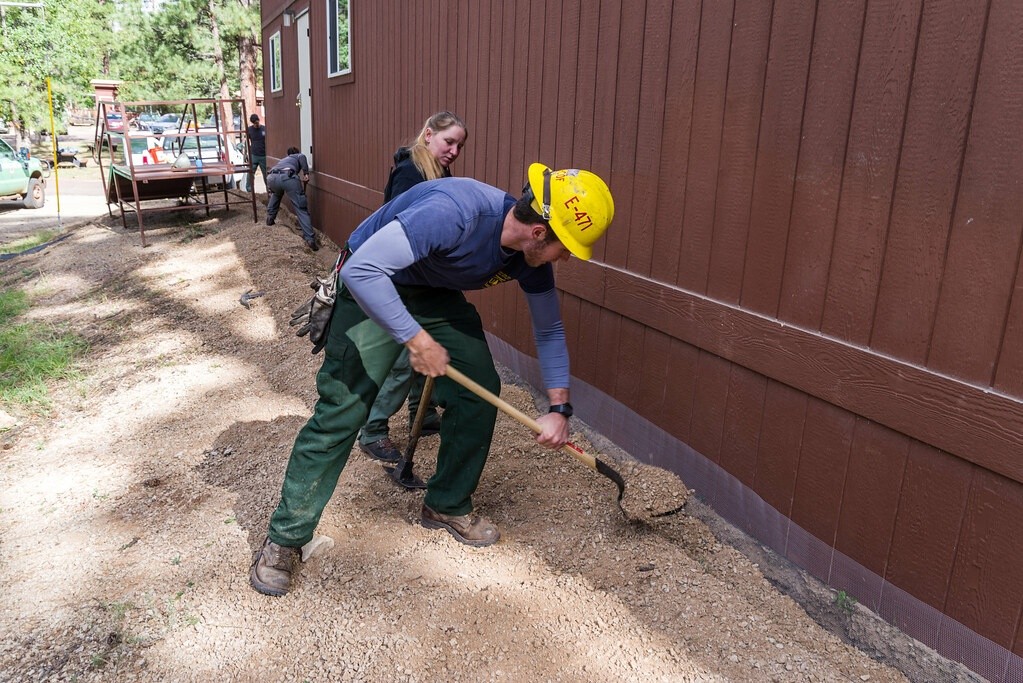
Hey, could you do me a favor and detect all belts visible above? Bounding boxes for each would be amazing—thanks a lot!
[270,170,286,174]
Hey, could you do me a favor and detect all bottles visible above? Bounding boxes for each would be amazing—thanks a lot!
[196,156,204,173]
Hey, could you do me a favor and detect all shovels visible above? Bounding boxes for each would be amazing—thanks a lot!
[294,177,307,230]
[446,365,689,522]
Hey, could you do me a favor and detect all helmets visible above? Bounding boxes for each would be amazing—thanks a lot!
[171,153,191,172]
[527,163,615,260]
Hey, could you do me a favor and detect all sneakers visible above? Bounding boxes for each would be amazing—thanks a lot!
[421,504,501,548]
[251,535,294,596]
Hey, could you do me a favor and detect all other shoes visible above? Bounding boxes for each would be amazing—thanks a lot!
[359,438,403,462]
[308,239,319,251]
[266,218,275,226]
[410,409,443,437]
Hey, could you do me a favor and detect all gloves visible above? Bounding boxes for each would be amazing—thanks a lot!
[289,276,338,355]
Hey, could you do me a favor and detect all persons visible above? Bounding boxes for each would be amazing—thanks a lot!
[266,146,320,251]
[251,162,615,598]
[243,113,267,192]
[357,111,469,463]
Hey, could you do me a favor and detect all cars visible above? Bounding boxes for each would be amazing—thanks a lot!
[0,138,46,209]
[15,109,252,181]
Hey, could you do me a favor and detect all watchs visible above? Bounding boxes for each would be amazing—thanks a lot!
[548,402,574,418]
